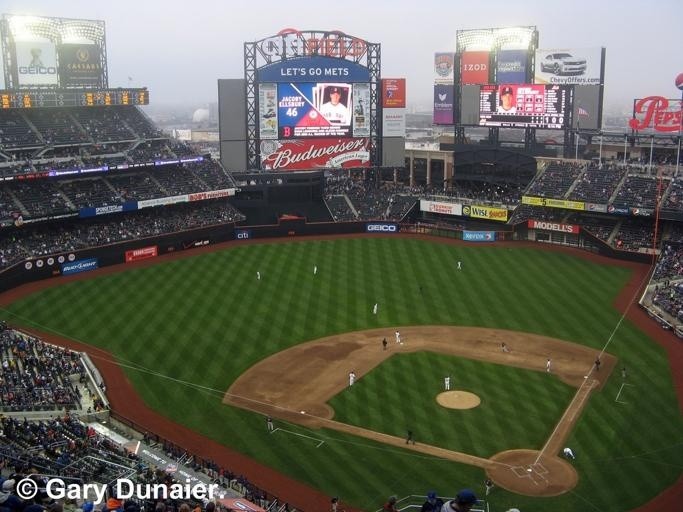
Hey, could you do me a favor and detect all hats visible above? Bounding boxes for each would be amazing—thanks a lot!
[502,87,513,95]
[82,501,93,512]
[428,491,435,501]
[329,86,341,94]
[456,489,480,505]
[2,479,15,490]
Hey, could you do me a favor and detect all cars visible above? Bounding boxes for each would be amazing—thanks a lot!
[540,53,587,75]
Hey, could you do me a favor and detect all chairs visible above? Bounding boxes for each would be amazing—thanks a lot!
[0,107,683,512]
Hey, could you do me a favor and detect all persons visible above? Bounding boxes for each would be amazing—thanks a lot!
[0,105,246,272]
[0,321,299,512]
[406,430,412,444]
[314,264,317,275]
[395,331,400,343]
[329,496,339,512]
[383,338,387,351]
[564,448,575,460]
[383,478,520,512]
[502,342,627,378]
[444,376,450,391]
[323,150,683,324]
[321,87,347,124]
[348,372,356,391]
[256,272,260,280]
[497,87,516,115]
